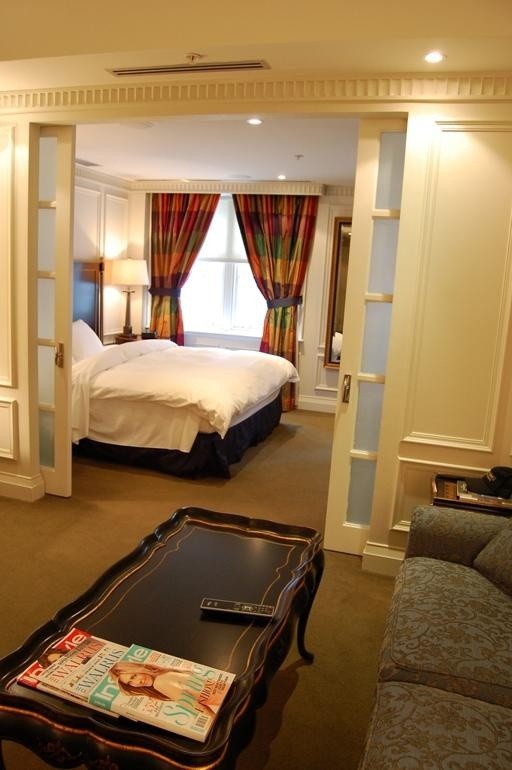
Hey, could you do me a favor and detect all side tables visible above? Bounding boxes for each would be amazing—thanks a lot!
[430,471,512,517]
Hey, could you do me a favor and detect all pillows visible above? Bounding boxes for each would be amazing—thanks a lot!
[470,518,512,592]
[72,319,104,362]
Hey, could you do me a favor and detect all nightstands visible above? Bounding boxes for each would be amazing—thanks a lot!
[116,335,171,343]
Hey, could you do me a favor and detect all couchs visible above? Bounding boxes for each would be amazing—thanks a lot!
[357,504,512,770]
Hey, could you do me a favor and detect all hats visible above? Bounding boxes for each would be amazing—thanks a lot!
[465,466,512,499]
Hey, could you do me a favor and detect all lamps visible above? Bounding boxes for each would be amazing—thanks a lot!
[113,257,151,339]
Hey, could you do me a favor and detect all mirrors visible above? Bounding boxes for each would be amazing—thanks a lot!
[323,217,352,370]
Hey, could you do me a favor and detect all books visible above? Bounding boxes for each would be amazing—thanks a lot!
[457,479,512,509]
[17,627,237,744]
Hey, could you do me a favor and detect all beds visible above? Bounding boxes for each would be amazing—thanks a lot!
[71,319,300,481]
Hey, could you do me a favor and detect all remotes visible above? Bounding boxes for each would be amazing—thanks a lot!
[201,598,277,618]
